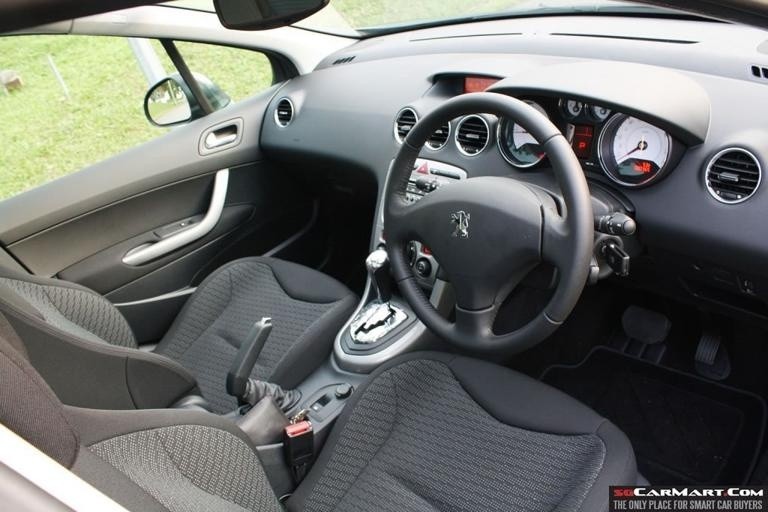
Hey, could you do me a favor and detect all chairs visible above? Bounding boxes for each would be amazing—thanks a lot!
[0,251,647,507]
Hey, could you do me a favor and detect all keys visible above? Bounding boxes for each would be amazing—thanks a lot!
[602,243,631,277]
